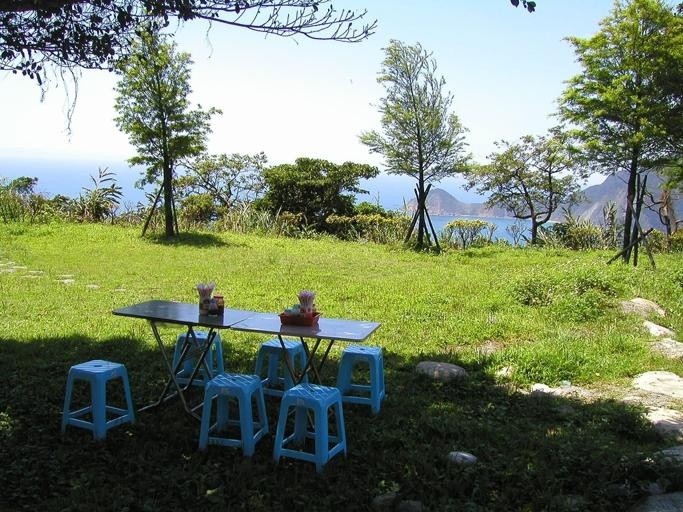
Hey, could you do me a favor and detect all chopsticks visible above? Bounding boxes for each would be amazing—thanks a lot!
[197,283,214,296]
[297,290,314,307]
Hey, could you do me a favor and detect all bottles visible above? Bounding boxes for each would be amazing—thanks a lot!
[285,304,316,317]
[209,295,224,315]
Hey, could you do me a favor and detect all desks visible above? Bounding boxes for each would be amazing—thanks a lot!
[230,310,382,433]
[112,300,257,423]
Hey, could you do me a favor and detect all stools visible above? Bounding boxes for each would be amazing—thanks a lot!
[168,330,224,391]
[254,339,309,397]
[61,360,137,441]
[337,345,385,414]
[199,372,273,458]
[273,382,347,473]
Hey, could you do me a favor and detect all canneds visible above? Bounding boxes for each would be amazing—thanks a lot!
[214,296,224,313]
